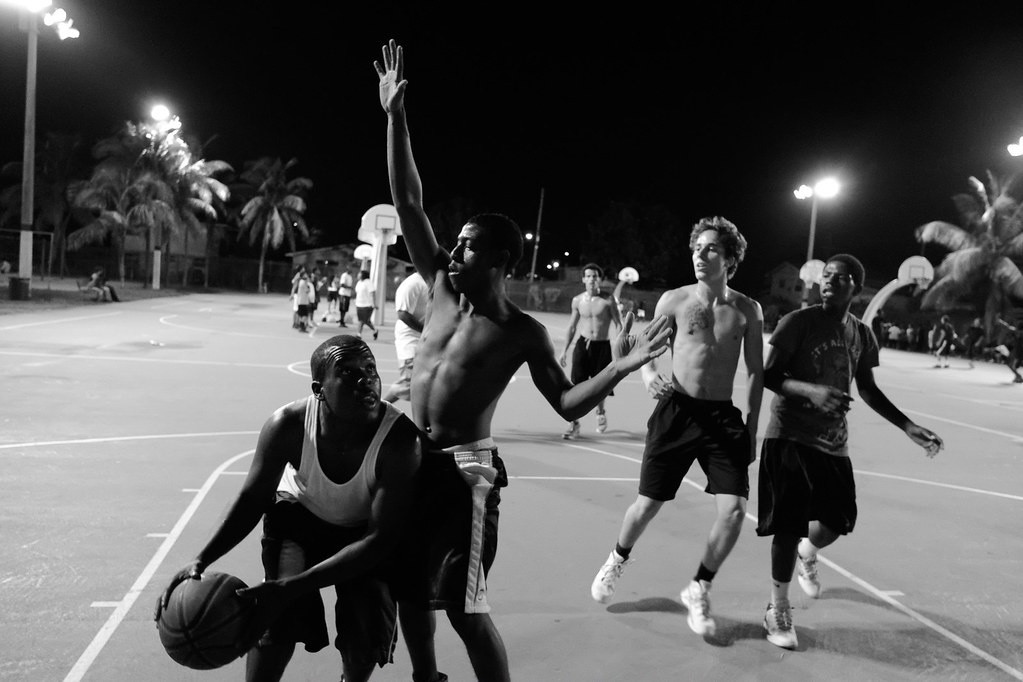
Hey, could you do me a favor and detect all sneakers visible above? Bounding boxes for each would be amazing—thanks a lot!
[761,602,798,649]
[796,552,822,598]
[596,409,607,432]
[561,423,580,439]
[681,578,717,637]
[590,549,630,604]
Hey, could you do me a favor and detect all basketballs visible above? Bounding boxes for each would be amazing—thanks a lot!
[157,571,257,671]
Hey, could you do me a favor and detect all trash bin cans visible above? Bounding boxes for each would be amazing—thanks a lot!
[7,277,31,300]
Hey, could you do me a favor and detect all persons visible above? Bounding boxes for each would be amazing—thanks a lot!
[877,310,1023,383]
[557,265,624,439]
[86,267,115,302]
[591,215,763,636]
[615,297,648,322]
[372,32,675,681]
[756,254,944,646]
[289,265,380,340]
[156,334,426,682]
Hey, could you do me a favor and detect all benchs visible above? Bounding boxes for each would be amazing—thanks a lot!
[76,278,99,301]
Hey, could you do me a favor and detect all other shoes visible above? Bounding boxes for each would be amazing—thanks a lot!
[373,330,378,340]
